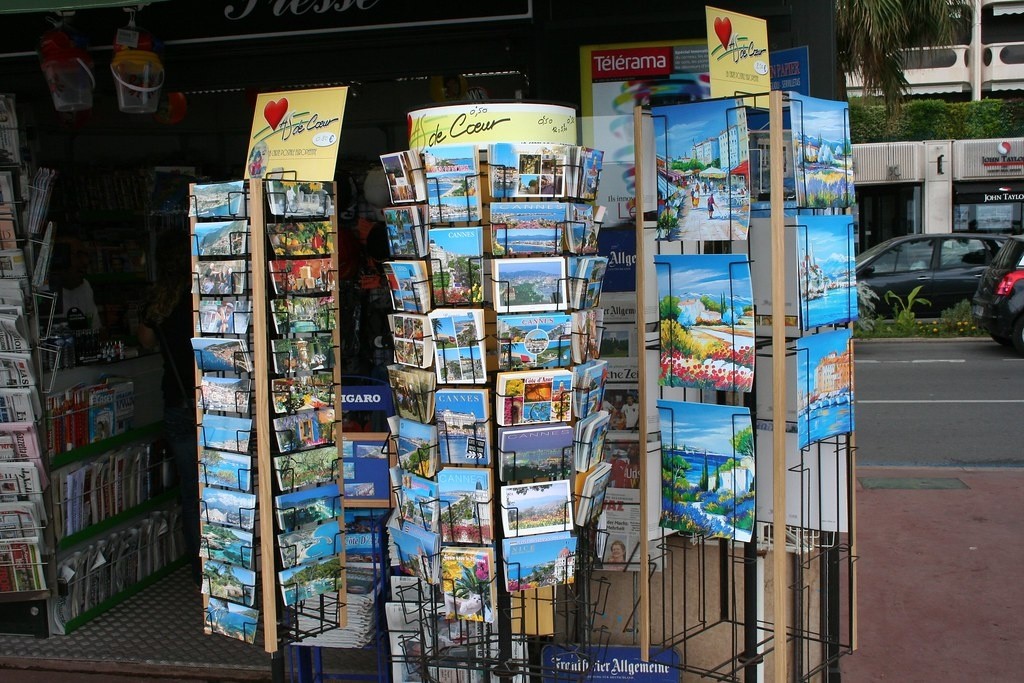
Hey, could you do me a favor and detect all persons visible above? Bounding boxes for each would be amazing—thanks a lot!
[36,238,108,334]
[137,227,201,607]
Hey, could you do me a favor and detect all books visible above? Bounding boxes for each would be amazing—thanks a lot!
[0,96,858,683]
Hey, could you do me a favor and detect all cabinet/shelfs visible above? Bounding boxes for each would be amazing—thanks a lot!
[1,62,860,683]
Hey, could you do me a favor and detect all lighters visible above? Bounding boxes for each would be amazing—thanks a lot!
[100,338,125,362]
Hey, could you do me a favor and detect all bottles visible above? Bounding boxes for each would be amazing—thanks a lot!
[39,322,77,371]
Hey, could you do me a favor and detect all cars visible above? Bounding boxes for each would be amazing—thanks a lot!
[970,233,1024,359]
[854,233,1011,320]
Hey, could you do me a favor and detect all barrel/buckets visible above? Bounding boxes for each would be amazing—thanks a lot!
[110,49,165,114]
[41,57,96,112]
